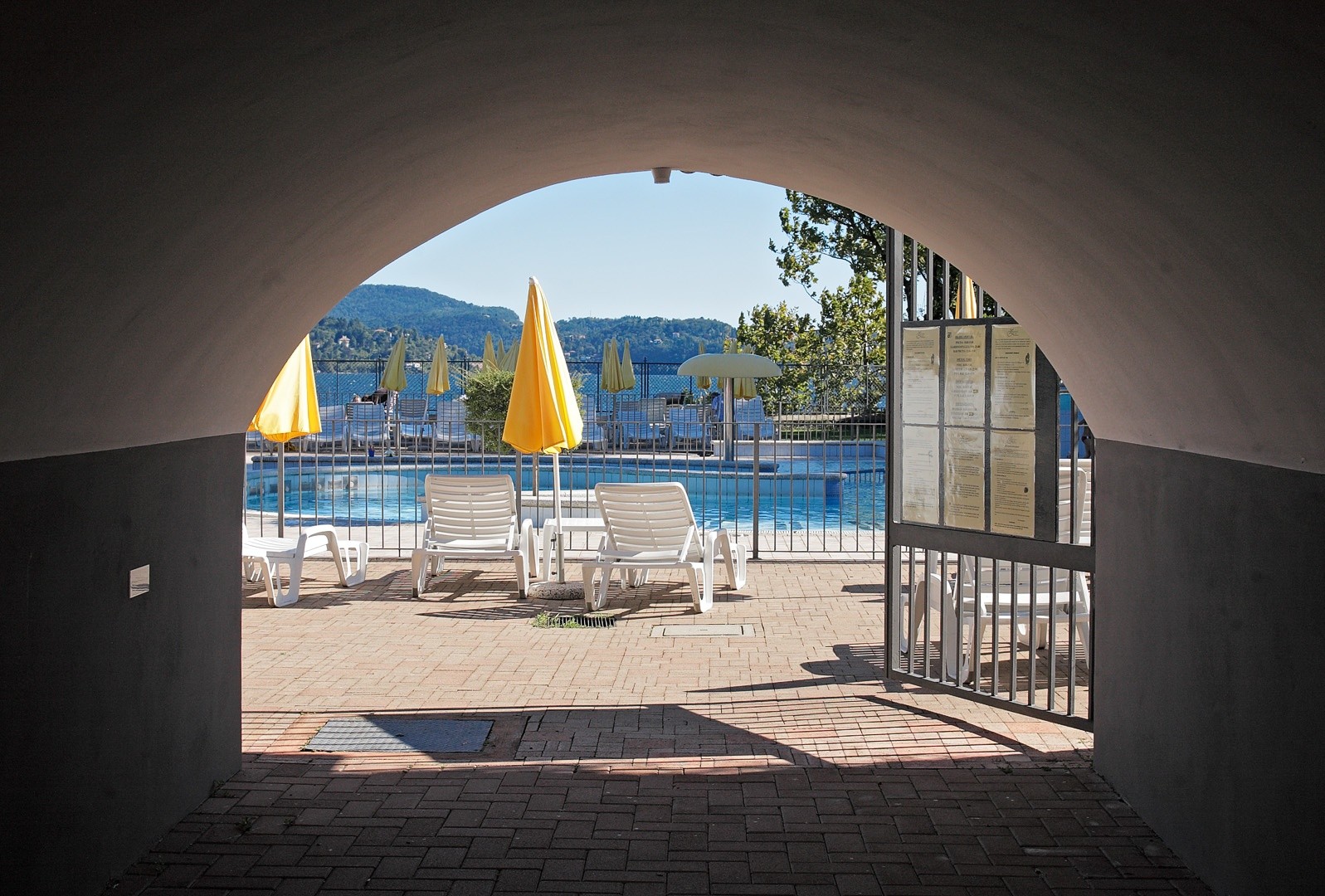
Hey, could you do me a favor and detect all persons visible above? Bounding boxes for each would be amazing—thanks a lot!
[1079,421,1095,459]
[351,389,389,405]
[712,392,730,439]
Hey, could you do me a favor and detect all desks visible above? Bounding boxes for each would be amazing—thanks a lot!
[542,517,626,589]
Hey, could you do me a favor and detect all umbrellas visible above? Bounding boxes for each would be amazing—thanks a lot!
[247,334,321,538]
[694,335,759,451]
[502,279,583,581]
[599,337,639,451]
[480,330,519,448]
[952,274,978,320]
[426,334,450,452]
[379,333,408,446]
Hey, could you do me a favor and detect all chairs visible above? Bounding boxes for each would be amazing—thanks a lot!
[734,396,774,439]
[583,480,747,613]
[247,399,482,454]
[412,473,539,597]
[901,456,1093,683]
[242,523,371,606]
[579,393,716,450]
